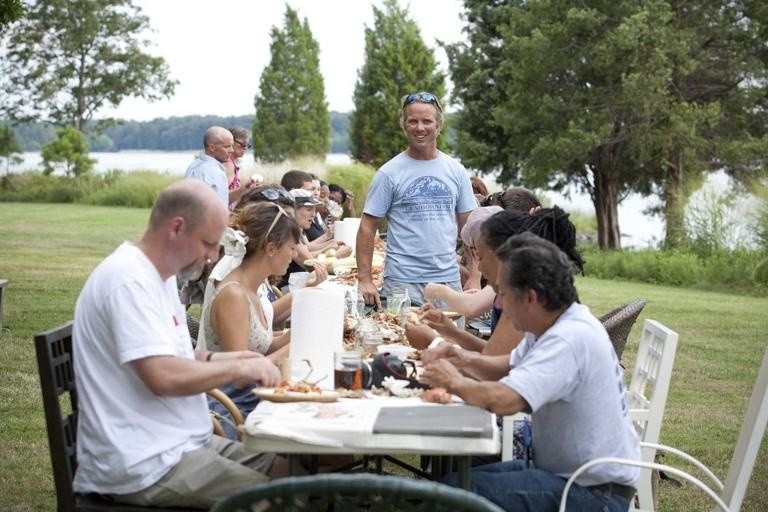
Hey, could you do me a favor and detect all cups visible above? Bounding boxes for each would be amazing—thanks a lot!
[330,289,413,393]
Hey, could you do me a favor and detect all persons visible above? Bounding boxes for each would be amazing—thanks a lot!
[183,125,235,213]
[353,89,483,330]
[71,177,314,512]
[417,229,642,512]
[225,124,250,213]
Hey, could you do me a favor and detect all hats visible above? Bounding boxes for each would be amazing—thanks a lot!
[288,187,323,209]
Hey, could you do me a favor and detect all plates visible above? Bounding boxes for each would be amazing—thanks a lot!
[411,308,463,321]
[248,387,335,404]
[301,258,379,272]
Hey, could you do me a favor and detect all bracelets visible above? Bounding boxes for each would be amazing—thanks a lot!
[205,350,218,365]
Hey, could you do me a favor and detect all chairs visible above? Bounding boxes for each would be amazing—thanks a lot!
[491,313,682,512]
[206,470,505,512]
[183,306,247,444]
[543,350,768,511]
[29,316,203,511]
[585,293,645,355]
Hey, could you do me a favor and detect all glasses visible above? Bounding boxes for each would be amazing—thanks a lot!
[234,137,254,151]
[255,187,296,206]
[400,91,443,111]
